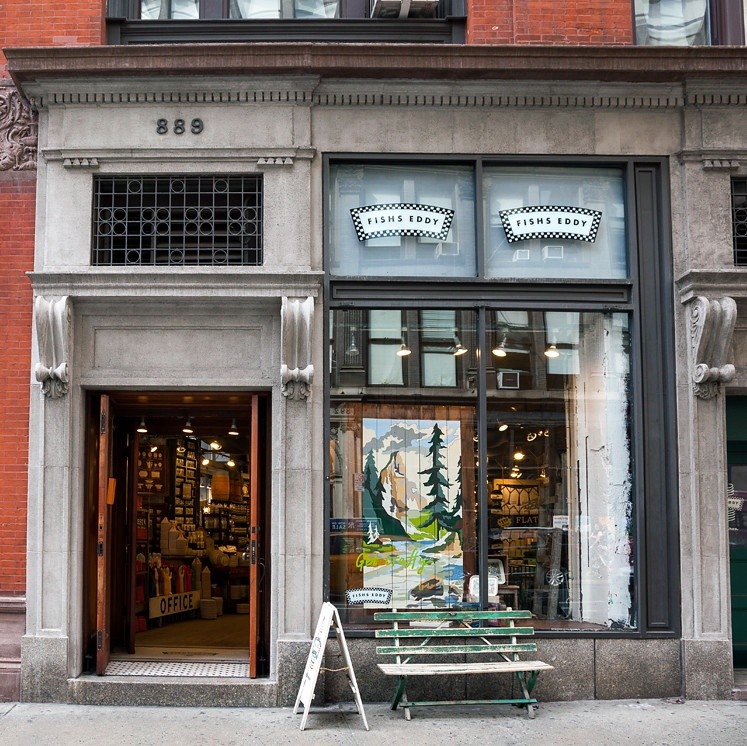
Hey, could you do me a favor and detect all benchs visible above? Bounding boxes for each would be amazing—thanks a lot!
[374,605,554,720]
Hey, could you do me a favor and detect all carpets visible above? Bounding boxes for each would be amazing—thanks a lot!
[100,660,250,678]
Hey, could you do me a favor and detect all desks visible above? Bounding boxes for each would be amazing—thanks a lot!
[224,572,249,608]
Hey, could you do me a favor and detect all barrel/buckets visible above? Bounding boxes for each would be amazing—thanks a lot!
[211,596,223,617]
[200,598,217,619]
[211,470,229,499]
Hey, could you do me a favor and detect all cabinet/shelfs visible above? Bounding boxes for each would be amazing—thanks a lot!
[135,432,250,555]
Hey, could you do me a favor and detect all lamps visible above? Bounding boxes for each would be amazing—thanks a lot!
[397,331,411,356]
[491,333,507,357]
[148,441,157,453]
[182,419,193,433]
[229,418,239,435]
[452,332,468,355]
[544,336,559,357]
[497,417,508,431]
[514,446,525,460]
[136,417,147,432]
[346,331,359,356]
